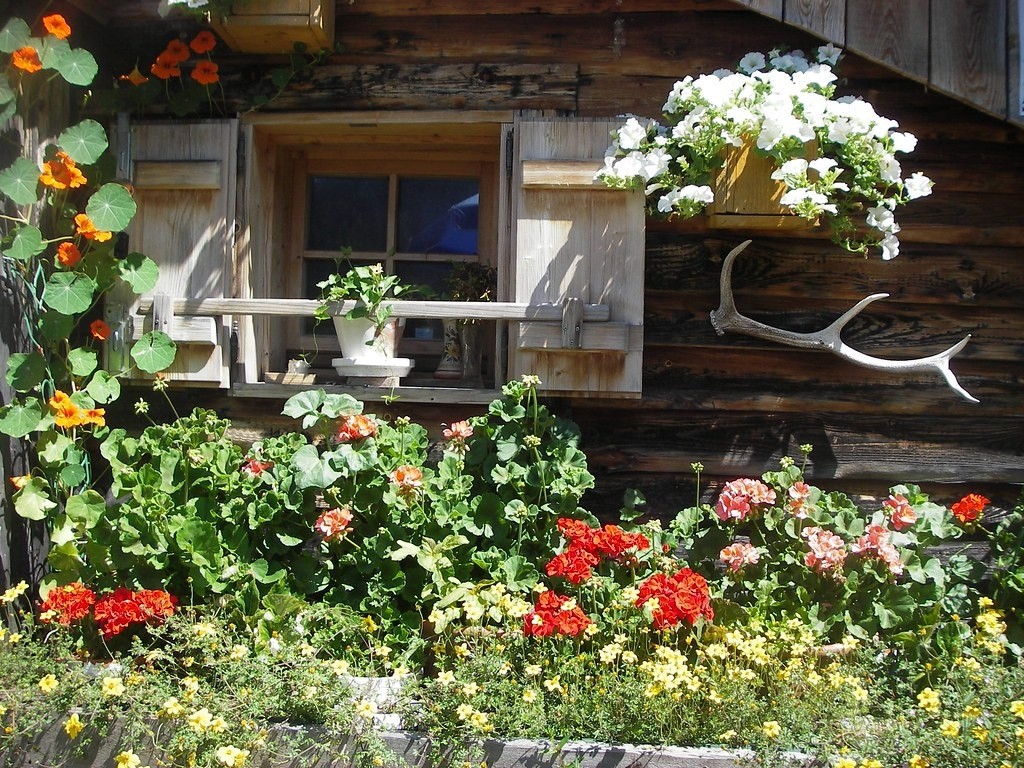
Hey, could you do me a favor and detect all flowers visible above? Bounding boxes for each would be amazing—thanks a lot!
[446,257,497,325]
[598,40,932,259]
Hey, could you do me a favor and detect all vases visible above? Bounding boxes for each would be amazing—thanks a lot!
[53,658,133,711]
[433,318,488,389]
[706,136,819,231]
[208,0,336,55]
[336,665,427,731]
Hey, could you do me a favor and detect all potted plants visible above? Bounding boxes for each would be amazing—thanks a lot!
[298,246,428,377]
